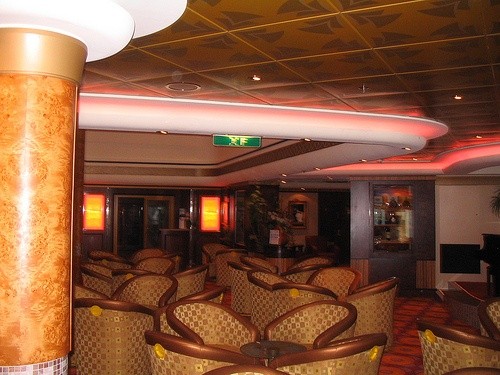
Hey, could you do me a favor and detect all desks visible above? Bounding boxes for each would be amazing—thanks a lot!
[240,341,307,363]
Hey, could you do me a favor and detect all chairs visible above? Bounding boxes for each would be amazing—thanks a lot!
[70,236,400,375]
[416,297,500,375]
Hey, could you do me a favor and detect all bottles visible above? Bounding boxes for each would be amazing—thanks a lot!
[402,197,410,207]
[397,196,402,207]
[378,210,382,218]
[386,227,391,241]
[390,212,397,224]
[389,197,397,207]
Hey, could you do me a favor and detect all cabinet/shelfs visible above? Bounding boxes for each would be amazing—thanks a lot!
[373,206,413,243]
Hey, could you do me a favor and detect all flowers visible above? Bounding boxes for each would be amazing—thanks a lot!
[268,202,295,247]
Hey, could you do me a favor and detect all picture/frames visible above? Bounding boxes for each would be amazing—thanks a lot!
[288,201,308,229]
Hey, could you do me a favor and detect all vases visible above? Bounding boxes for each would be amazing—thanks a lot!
[273,223,286,245]
